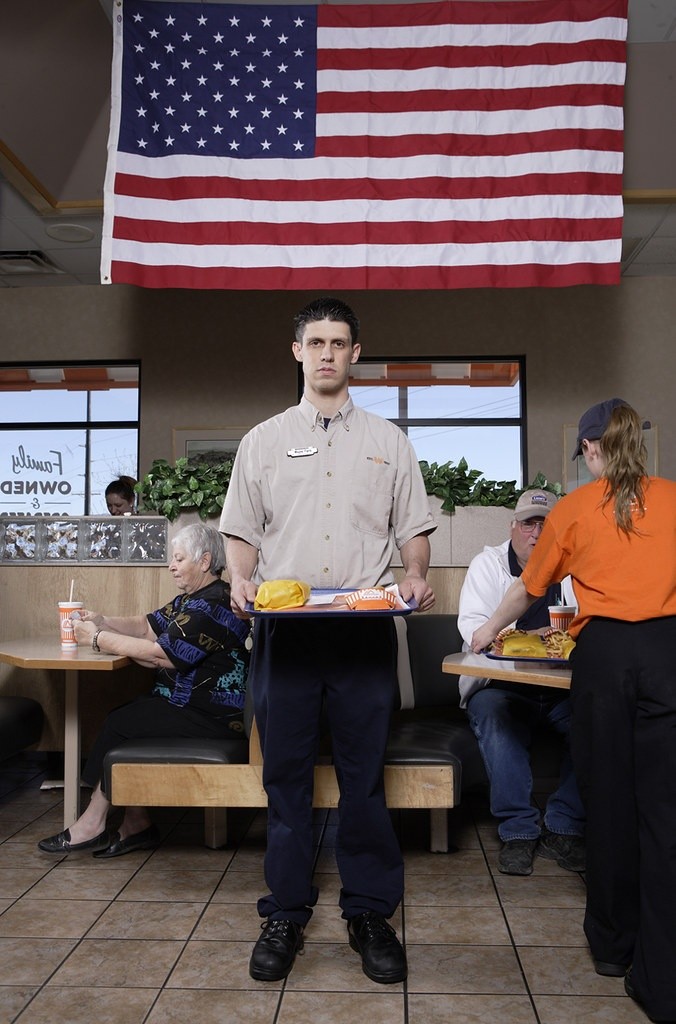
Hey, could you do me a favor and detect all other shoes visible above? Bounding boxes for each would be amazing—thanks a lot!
[593,954,630,977]
[624,964,636,999]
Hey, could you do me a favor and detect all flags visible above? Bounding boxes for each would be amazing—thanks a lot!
[100,0,628,291]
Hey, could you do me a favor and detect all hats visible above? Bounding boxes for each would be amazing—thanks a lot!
[571,398,625,461]
[514,489,558,522]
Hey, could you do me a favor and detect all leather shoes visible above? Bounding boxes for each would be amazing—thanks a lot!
[248,920,304,981]
[92,823,161,858]
[38,828,111,854]
[346,911,408,983]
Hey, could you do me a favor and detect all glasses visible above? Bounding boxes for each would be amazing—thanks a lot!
[521,521,544,533]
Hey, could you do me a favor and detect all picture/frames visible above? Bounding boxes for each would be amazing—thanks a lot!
[562,423,595,493]
[170,425,261,469]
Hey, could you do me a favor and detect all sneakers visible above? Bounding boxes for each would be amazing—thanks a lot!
[538,823,586,870]
[497,838,539,874]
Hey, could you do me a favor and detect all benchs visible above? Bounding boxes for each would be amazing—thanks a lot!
[274,570,569,855]
[96,586,265,857]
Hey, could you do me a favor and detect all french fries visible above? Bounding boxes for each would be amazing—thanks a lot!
[492,630,524,650]
[543,630,573,655]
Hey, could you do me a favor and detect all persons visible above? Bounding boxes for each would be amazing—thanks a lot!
[219,300,437,985]
[456,398,676,1022]
[40,523,252,857]
[105,476,138,516]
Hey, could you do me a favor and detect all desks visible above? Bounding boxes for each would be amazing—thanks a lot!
[443,648,571,692]
[0,634,132,850]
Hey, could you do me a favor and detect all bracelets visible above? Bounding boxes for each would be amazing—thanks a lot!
[92,628,102,652]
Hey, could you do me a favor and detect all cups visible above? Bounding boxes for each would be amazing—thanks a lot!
[548,605,577,631]
[58,601,83,647]
